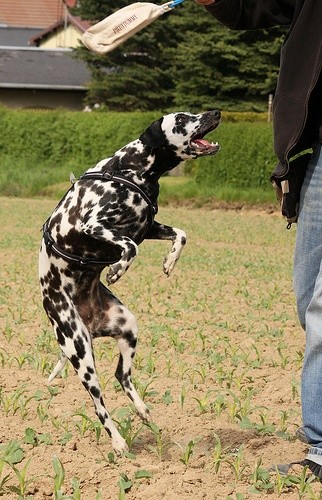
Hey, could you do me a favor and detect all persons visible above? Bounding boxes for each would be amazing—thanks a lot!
[191,0,322,482]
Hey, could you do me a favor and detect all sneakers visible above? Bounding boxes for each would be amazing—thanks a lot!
[263,458,322,483]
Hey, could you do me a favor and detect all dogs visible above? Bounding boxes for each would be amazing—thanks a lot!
[37,107,222,458]
